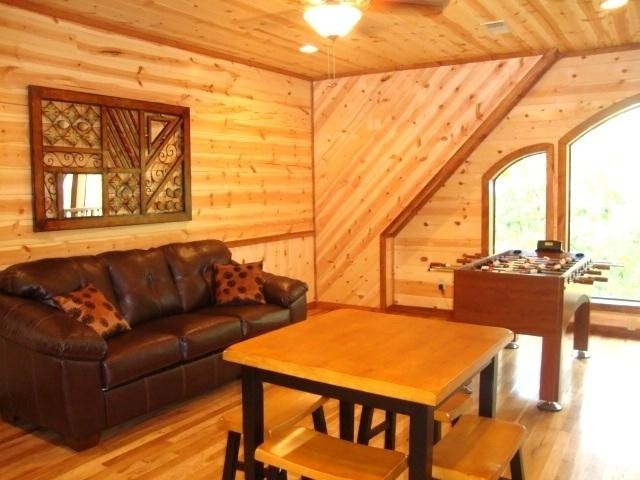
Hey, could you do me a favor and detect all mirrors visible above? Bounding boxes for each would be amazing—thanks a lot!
[58,171,103,219]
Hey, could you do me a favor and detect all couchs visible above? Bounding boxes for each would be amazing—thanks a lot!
[0,240,308,449]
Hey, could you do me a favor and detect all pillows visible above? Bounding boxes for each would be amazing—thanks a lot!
[53,284,132,337]
[213,259,268,307]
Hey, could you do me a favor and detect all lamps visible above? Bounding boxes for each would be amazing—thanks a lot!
[302,0,366,87]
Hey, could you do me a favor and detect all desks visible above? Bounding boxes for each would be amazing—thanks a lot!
[223,307,514,480]
